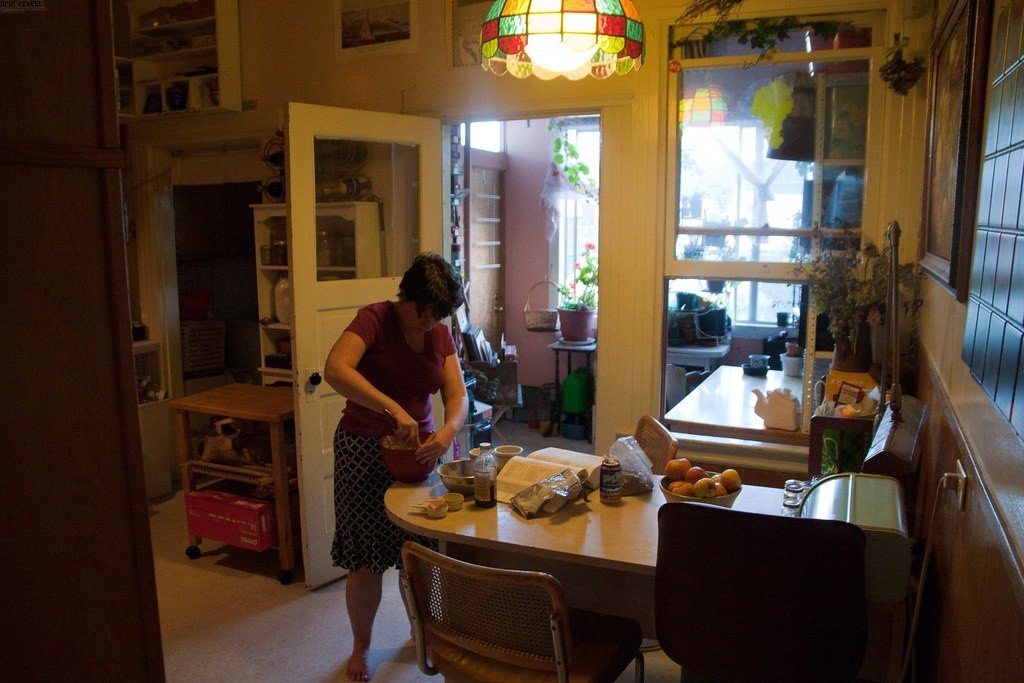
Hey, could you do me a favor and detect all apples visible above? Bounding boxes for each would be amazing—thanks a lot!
[664,457,741,499]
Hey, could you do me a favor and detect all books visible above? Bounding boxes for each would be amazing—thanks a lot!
[496,446,611,514]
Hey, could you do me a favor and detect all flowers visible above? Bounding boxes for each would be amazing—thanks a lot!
[556,244,599,311]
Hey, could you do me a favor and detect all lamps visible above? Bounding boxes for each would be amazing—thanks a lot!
[477,0,646,81]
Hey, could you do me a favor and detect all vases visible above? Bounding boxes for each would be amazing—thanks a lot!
[556,306,596,342]
[831,319,873,373]
[708,280,726,293]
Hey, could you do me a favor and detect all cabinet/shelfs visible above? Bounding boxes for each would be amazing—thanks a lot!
[248,202,383,387]
[115,0,241,124]
[168,382,296,585]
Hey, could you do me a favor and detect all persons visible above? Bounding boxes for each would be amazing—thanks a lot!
[323,251,469,682]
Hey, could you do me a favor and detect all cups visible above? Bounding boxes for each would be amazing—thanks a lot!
[748,354,770,368]
[780,353,803,377]
[469,447,480,460]
[494,445,523,475]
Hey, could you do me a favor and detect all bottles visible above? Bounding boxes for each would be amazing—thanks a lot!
[259,151,285,168]
[261,230,354,325]
[323,143,369,196]
[165,82,189,111]
[474,442,497,508]
[781,476,819,518]
[257,182,285,199]
[562,368,588,413]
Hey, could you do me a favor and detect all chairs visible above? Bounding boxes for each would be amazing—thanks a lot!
[400,541,644,683]
[655,501,869,683]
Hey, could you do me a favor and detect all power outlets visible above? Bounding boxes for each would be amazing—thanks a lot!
[955,460,967,511]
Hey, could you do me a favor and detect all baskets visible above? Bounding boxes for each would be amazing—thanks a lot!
[521,282,558,331]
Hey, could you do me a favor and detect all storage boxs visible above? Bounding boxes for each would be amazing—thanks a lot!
[185,478,278,553]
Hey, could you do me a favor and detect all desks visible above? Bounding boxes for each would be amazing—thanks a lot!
[664,344,731,374]
[663,365,811,492]
[547,341,596,441]
[384,461,785,641]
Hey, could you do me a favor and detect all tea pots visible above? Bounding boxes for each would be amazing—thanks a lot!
[751,388,801,431]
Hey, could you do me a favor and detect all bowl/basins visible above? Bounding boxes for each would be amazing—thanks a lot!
[379,431,439,483]
[740,364,770,375]
[436,459,474,496]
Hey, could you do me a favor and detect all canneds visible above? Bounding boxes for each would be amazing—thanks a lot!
[599,460,621,504]
[261,241,288,265]
